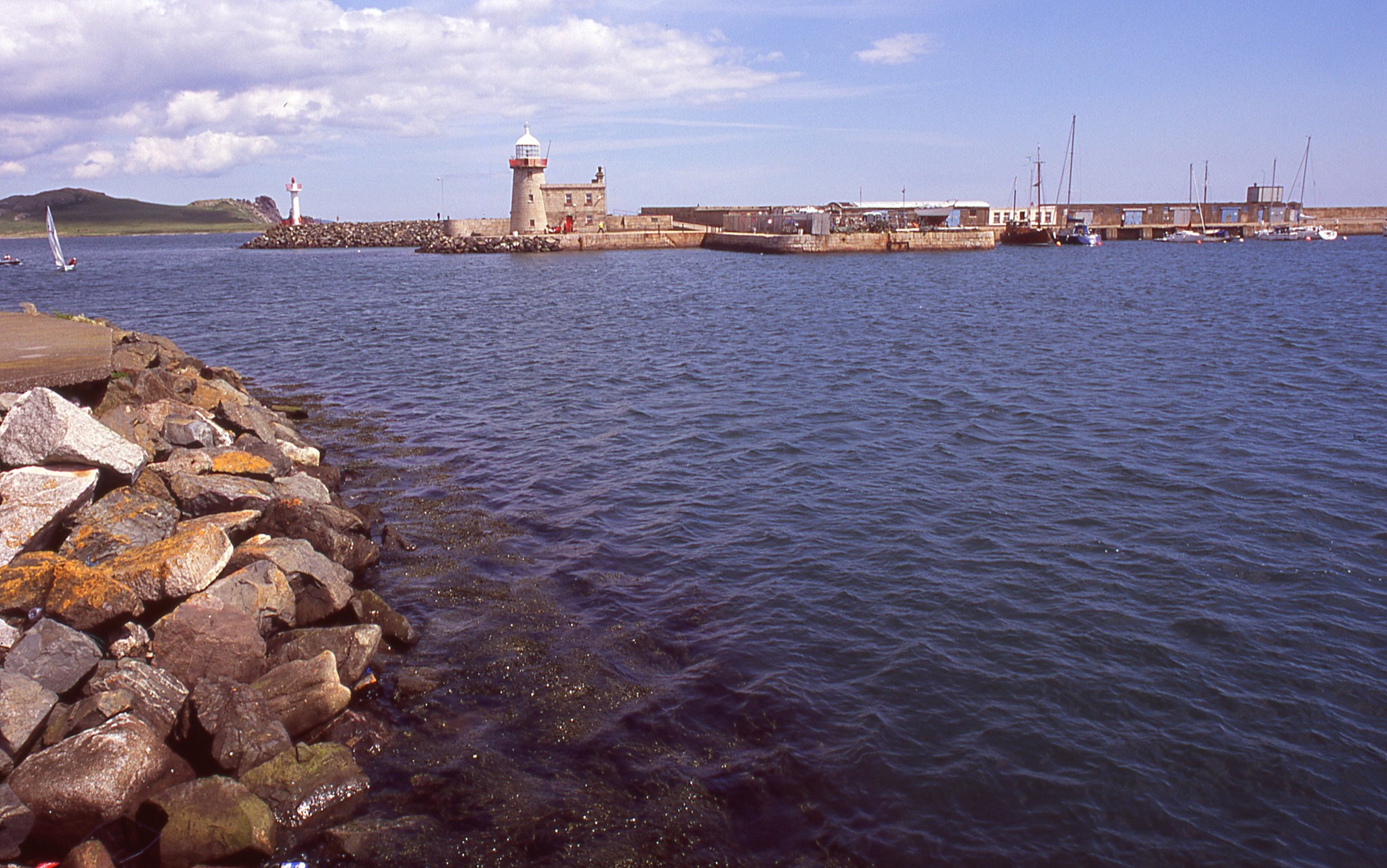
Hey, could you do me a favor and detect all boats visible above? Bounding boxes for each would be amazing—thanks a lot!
[0,253,22,265]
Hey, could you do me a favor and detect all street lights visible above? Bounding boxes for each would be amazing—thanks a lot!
[436,176,445,221]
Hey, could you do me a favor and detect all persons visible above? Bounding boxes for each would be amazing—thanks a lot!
[4,254,11,260]
[597,221,604,233]
[68,257,77,266]
[564,221,567,235]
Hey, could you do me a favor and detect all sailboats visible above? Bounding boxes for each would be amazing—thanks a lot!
[998,142,1054,246]
[46,205,77,271]
[1049,115,1102,245]
[1152,159,1241,241]
[1252,137,1338,241]
